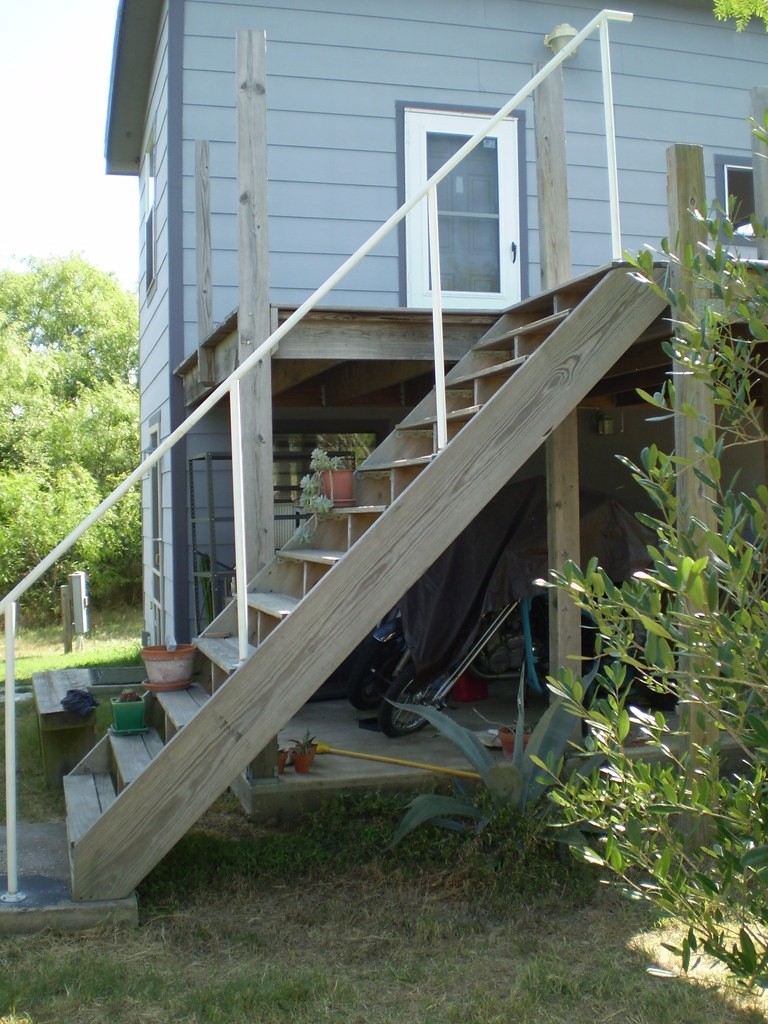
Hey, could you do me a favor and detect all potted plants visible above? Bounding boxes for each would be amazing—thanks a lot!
[277,744,289,773]
[287,727,318,774]
[110,689,145,730]
[500,720,538,760]
[293,448,353,547]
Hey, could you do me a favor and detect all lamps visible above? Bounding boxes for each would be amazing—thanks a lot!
[597,410,613,435]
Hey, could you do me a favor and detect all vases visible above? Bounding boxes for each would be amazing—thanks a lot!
[141,644,197,685]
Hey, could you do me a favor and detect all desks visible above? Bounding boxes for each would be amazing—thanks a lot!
[32,669,97,789]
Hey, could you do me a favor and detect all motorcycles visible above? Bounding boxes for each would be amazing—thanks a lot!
[346,477,677,738]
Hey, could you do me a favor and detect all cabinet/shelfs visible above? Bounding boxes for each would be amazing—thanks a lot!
[187,450,356,635]
